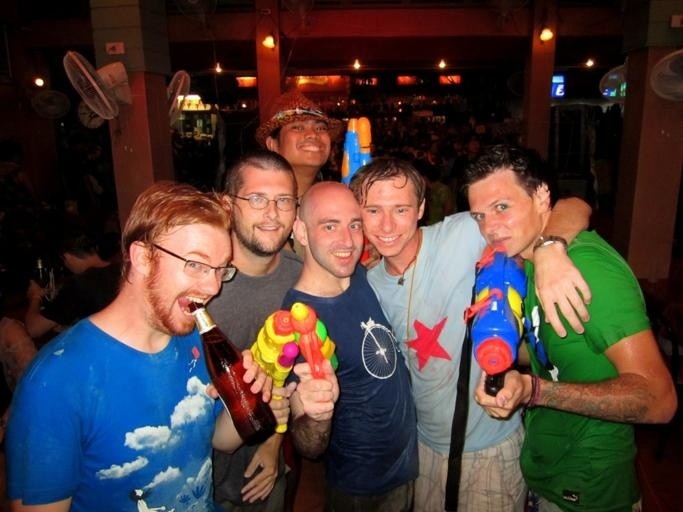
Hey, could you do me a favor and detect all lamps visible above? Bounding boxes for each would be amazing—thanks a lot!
[262,33,276,49]
[539,16,553,42]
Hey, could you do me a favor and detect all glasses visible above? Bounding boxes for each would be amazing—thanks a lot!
[227,193,294,212]
[151,240,237,283]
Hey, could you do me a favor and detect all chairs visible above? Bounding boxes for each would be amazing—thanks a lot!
[635,278,682,462]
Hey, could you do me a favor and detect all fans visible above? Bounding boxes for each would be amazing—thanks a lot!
[649,49,683,102]
[29,50,192,134]
[598,65,626,105]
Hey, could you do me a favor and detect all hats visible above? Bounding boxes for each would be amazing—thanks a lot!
[255,91,344,150]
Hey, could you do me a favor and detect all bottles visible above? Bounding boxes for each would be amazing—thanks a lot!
[188,301,277,447]
[35,258,51,308]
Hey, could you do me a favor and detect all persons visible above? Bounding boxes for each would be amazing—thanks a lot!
[2,83,677,512]
